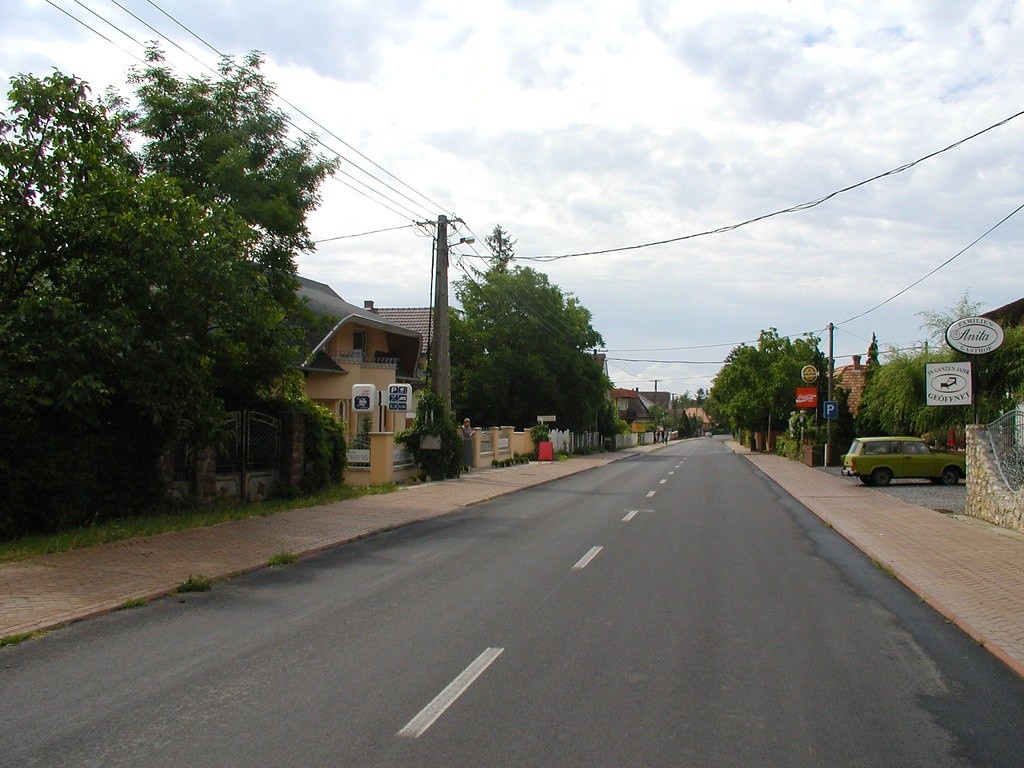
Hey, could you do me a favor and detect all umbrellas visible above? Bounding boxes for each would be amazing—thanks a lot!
[947,427,956,446]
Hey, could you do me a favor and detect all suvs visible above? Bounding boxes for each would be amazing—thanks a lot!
[840,435,966,487]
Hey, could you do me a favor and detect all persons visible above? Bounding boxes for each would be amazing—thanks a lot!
[462,418,475,466]
[656,428,668,444]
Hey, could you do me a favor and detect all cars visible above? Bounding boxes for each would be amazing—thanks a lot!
[705,431,712,437]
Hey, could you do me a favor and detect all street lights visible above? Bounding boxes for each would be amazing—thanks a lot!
[434,236,476,431]
